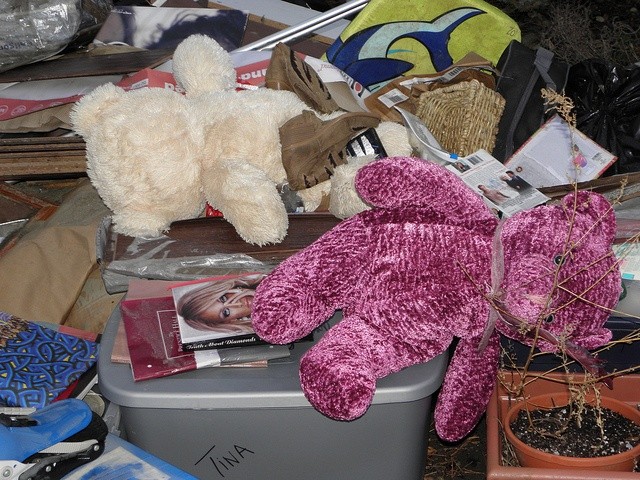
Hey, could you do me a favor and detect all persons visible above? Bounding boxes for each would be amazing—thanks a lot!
[177,280,263,336]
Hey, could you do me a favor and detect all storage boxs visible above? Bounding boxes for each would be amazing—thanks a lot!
[97,293,450,480]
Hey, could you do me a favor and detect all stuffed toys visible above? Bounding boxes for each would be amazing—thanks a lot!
[69,35,415,246]
[250,155,621,441]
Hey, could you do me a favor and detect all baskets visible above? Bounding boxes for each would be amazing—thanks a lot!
[415,79,506,158]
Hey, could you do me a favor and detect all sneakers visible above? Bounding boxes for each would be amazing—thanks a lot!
[279,111,380,190]
[265,42,336,112]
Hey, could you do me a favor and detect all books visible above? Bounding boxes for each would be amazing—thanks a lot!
[0,312,102,415]
[118,296,291,381]
[111,280,267,366]
[171,275,313,352]
[445,147,550,220]
[130,4,246,53]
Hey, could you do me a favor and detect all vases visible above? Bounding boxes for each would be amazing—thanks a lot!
[503,390,640,470]
[486,372,640,480]
